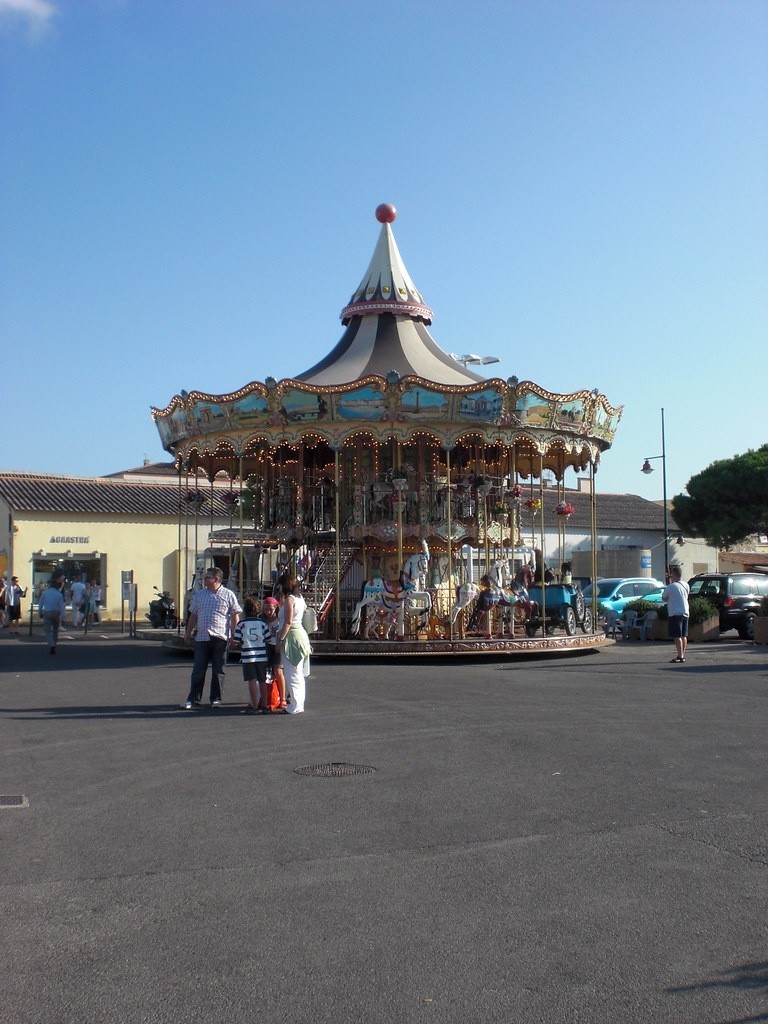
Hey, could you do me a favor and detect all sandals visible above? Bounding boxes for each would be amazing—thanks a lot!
[670,657,686,663]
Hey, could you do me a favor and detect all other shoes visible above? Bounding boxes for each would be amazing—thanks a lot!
[258,703,270,714]
[239,704,259,715]
[15,632,18,634]
[179,700,201,709]
[10,632,12,634]
[285,704,303,714]
[275,704,288,714]
[212,700,221,708]
[50,647,55,655]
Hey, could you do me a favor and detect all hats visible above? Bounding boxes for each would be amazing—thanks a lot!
[263,597,278,605]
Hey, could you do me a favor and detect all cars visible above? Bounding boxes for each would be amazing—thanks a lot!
[633,586,700,606]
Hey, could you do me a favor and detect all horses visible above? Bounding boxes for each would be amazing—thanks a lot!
[444,558,539,630]
[351,554,432,641]
[181,559,237,627]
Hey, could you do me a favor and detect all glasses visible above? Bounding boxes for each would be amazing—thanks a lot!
[204,573,220,581]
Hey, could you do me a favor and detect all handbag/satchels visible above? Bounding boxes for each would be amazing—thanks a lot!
[301,607,318,634]
[258,677,281,710]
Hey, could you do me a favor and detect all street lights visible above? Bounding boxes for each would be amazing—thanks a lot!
[639,407,687,585]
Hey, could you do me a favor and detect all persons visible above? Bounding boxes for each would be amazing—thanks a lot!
[661,567,690,663]
[180,567,314,714]
[0,572,110,655]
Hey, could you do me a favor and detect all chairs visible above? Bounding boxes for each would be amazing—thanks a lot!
[603,610,658,642]
[707,586,720,594]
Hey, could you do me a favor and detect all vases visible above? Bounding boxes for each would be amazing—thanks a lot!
[479,485,491,496]
[226,503,236,514]
[189,502,202,512]
[529,509,537,515]
[557,514,570,521]
[392,479,406,490]
[516,493,522,497]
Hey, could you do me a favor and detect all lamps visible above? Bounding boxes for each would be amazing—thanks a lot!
[449,352,500,369]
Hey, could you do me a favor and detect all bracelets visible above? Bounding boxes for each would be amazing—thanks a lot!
[278,637,283,642]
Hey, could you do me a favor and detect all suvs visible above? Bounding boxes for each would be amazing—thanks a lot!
[580,577,664,621]
[686,572,768,640]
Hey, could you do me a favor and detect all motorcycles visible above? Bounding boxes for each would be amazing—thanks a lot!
[144,586,179,630]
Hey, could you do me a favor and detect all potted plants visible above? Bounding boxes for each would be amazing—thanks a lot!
[753,596,768,646]
[622,595,720,642]
[588,601,608,628]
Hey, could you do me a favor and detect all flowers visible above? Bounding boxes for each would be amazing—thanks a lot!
[385,463,415,483]
[183,491,207,502]
[473,473,493,489]
[513,485,525,494]
[553,501,574,514]
[220,493,239,504]
[524,499,541,510]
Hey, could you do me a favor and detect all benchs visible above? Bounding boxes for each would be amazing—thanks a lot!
[529,584,577,607]
